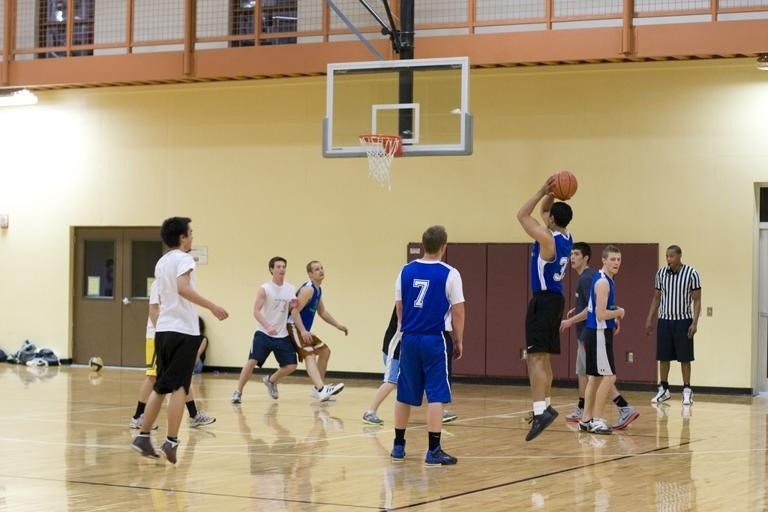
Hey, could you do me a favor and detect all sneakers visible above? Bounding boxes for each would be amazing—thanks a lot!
[361,410,384,426]
[188,411,217,429]
[230,388,243,405]
[130,434,161,460]
[424,468,462,487]
[362,426,385,439]
[187,430,216,444]
[681,406,693,418]
[564,423,581,438]
[579,418,611,434]
[441,428,457,442]
[314,404,344,438]
[231,405,243,416]
[649,385,672,403]
[159,438,180,467]
[129,413,159,431]
[680,386,693,405]
[389,437,407,463]
[650,404,671,417]
[612,431,641,454]
[310,382,345,403]
[390,464,410,492]
[424,445,458,467]
[129,460,159,496]
[611,402,640,431]
[564,406,584,422]
[262,374,279,400]
[525,405,559,442]
[577,434,610,449]
[263,401,280,427]
[441,409,458,423]
[160,465,178,497]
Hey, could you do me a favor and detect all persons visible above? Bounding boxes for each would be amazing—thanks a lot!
[643,245,702,404]
[390,226,467,465]
[362,305,457,425]
[192,316,208,375]
[288,260,348,401]
[516,176,573,441]
[579,245,626,434]
[130,276,217,429]
[560,242,640,430]
[231,257,300,403]
[129,218,229,466]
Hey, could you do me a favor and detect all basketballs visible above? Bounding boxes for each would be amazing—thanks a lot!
[552,170,578,201]
[89,357,102,371]
[88,372,103,386]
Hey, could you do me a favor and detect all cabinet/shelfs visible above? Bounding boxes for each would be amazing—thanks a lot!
[407,242,447,264]
[568,242,660,387]
[486,242,529,379]
[528,242,569,383]
[449,244,486,381]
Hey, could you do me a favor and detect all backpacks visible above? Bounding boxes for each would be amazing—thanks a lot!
[0,362,59,391]
[0,339,60,368]
